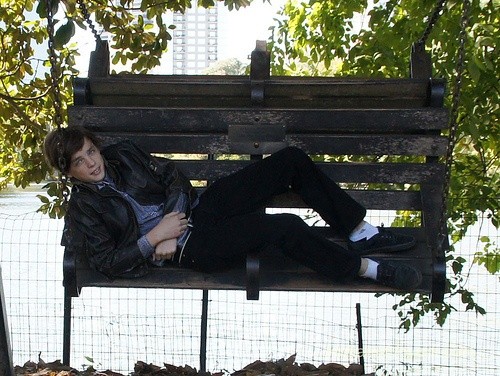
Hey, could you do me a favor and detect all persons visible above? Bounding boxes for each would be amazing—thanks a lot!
[46,127,423,300]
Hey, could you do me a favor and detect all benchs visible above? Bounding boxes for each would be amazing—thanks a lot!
[61,41,451,305]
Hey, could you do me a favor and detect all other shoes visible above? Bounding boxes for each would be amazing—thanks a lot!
[348,226,415,254]
[376,261,421,290]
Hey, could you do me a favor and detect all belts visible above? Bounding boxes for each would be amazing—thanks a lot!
[172,217,194,264]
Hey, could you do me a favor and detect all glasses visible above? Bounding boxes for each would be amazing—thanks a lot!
[57,127,66,174]
